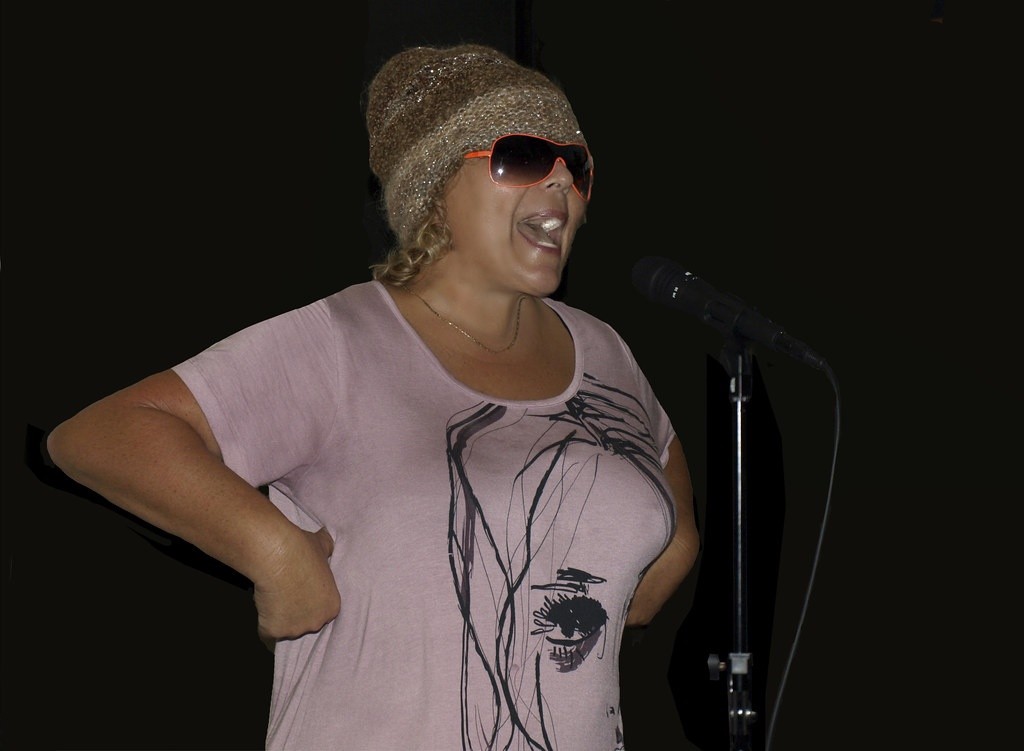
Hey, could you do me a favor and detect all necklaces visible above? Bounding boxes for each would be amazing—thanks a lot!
[402,285,527,355]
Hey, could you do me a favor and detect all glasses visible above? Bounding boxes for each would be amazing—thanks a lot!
[464,135,595,202]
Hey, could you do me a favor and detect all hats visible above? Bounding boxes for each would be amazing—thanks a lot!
[367,45,587,242]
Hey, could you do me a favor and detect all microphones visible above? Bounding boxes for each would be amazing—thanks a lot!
[633,252,824,375]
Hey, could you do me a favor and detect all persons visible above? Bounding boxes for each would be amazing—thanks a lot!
[47,44,699,751]
